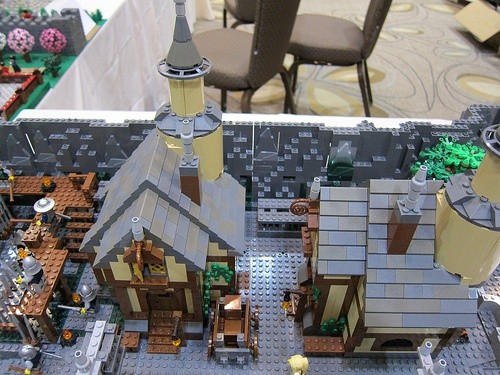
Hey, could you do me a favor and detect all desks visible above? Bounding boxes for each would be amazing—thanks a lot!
[35,0,216,112]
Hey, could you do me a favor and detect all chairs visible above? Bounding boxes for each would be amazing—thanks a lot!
[284,0,393,117]
[222,0,258,29]
[190,0,301,115]
